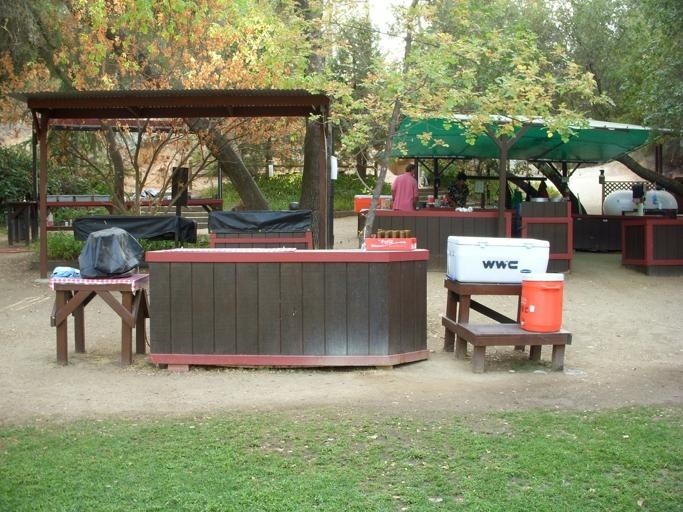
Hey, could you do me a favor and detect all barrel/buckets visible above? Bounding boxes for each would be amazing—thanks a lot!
[520,273,563,333]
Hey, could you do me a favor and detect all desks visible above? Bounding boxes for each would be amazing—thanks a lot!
[51,272,149,369]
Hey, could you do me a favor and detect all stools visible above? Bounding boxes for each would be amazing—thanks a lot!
[442,277,572,373]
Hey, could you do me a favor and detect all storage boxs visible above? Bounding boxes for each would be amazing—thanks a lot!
[446,235,551,284]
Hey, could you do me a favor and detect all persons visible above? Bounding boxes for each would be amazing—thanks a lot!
[392,163,419,212]
[447,172,469,208]
